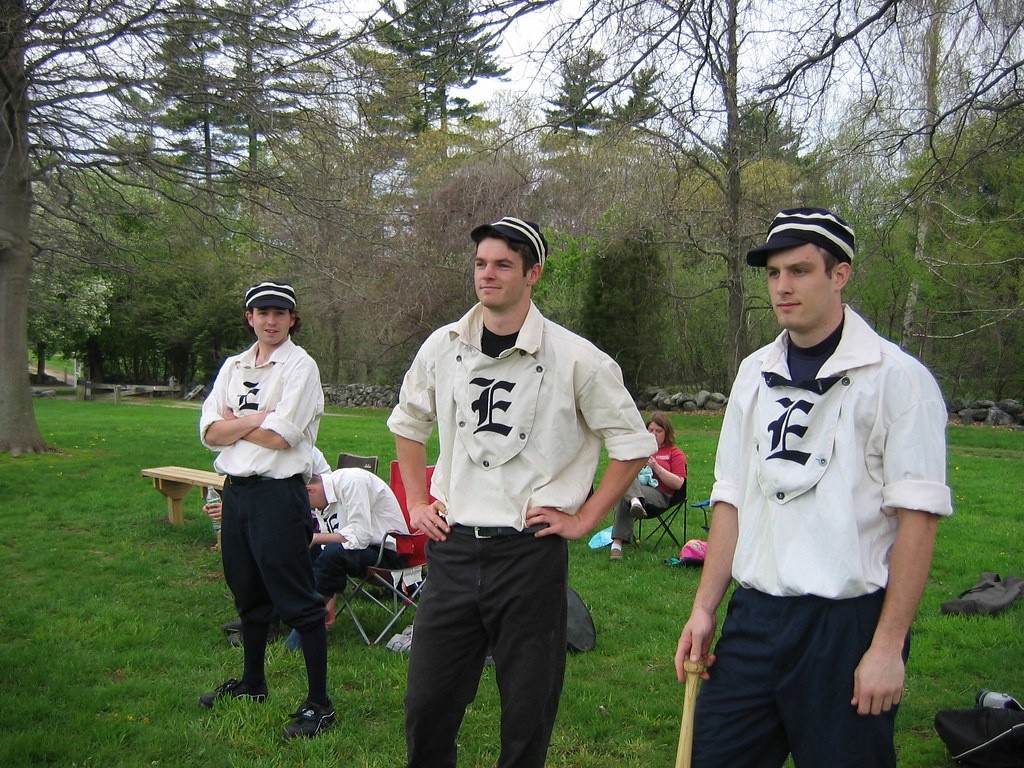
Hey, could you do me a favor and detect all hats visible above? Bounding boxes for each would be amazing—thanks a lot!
[746,208,856,267]
[470,214,549,270]
[244,281,297,311]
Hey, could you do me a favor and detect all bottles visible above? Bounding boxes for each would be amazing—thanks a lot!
[206,484,225,532]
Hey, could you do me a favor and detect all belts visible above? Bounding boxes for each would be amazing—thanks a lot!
[227,474,273,484]
[438,517,549,538]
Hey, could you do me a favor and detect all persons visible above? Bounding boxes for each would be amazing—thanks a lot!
[386,216,659,768]
[199,281,338,739]
[674,207,953,768]
[284,467,413,650]
[203,445,333,645]
[610,411,686,560]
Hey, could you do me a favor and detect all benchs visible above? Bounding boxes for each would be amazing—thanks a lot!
[140,466,227,526]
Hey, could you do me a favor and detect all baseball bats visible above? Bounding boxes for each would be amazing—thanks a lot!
[674,658,706,768]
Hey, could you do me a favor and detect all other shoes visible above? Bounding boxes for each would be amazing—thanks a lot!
[610,543,622,559]
[283,629,303,651]
[630,504,647,519]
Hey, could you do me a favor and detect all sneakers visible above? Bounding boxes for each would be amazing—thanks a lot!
[200,678,268,708]
[284,699,336,739]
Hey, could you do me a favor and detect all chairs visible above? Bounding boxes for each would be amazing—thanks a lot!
[337,454,378,477]
[633,464,688,552]
[336,460,436,644]
